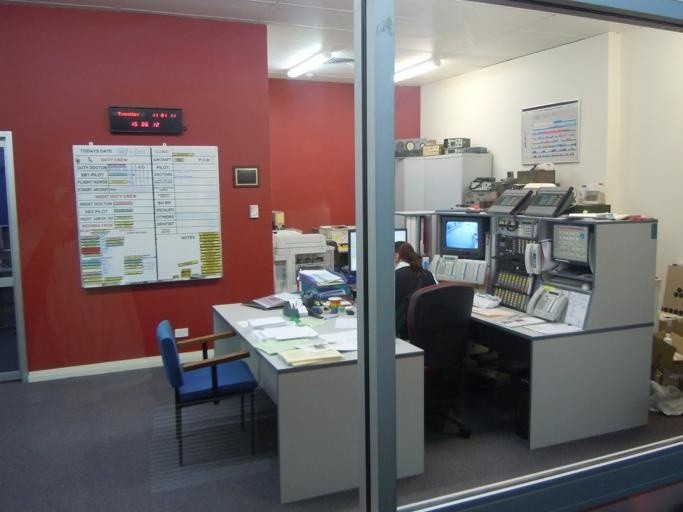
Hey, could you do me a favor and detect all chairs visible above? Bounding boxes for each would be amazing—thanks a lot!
[156,318,260,467]
[407,281,475,439]
[326,240,349,268]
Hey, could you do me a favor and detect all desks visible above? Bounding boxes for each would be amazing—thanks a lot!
[213,304,359,505]
[469,294,654,450]
[286,294,424,483]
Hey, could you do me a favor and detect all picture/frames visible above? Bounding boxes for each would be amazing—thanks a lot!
[233,165,259,187]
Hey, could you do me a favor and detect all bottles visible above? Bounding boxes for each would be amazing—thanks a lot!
[597,183,606,204]
[579,185,587,204]
[662,333,672,346]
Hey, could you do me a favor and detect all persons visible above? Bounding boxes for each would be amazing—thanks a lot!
[395,241,438,340]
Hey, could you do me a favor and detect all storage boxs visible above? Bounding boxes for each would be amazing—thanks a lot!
[650,264,683,386]
[318,225,348,245]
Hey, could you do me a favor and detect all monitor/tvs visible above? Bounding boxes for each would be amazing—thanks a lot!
[551,222,589,275]
[441,215,489,260]
[347,228,408,275]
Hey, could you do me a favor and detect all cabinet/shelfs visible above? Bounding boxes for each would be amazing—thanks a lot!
[273,244,335,294]
[394,153,491,212]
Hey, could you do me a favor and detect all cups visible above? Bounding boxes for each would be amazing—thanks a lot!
[328,296,342,314]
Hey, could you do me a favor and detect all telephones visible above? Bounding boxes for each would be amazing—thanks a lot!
[524,243,542,275]
[526,285,569,322]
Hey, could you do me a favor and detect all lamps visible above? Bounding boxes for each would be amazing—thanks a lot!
[288,51,333,78]
[394,58,441,83]
